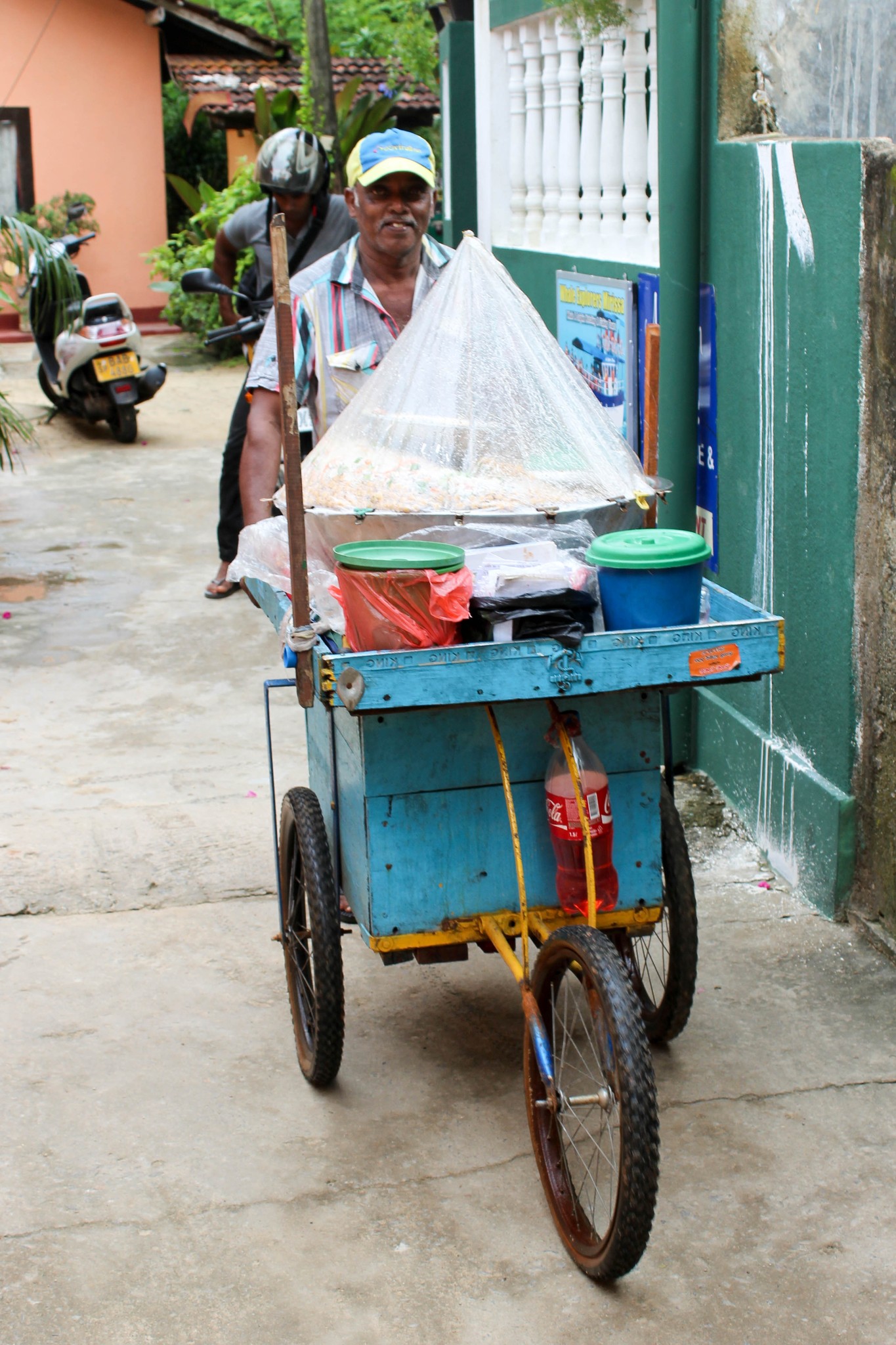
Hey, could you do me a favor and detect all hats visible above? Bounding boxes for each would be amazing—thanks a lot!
[346,127,435,194]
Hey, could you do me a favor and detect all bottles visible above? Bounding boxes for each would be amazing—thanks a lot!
[699,585,710,625]
[545,709,619,916]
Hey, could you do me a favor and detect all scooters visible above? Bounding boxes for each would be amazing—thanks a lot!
[179,268,315,516]
[4,201,168,444]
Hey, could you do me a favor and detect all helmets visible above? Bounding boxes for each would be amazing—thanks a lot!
[255,128,331,204]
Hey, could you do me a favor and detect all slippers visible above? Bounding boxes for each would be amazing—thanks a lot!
[204,577,240,599]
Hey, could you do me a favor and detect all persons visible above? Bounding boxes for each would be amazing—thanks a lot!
[238,130,455,607]
[205,127,358,599]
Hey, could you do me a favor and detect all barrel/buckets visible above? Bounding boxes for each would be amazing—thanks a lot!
[333,540,465,652]
[584,527,713,631]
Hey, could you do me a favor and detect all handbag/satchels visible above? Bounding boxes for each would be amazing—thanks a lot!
[237,262,257,319]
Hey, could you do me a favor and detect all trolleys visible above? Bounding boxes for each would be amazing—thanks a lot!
[247,546,788,1285]
